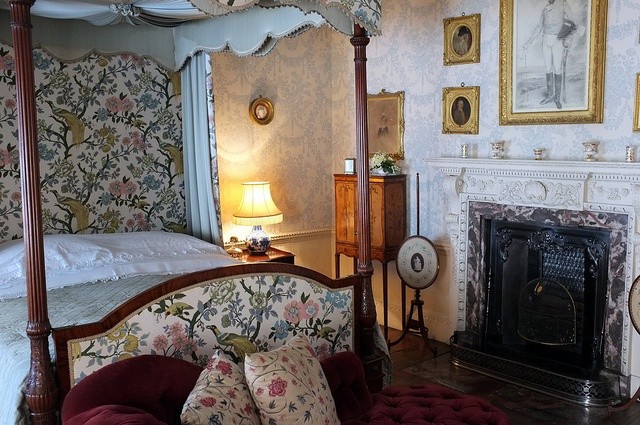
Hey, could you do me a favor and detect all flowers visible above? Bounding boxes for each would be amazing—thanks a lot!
[100,127,114,153]
[11,150,20,164]
[131,168,143,178]
[209,280,236,306]
[271,319,289,340]
[283,299,319,323]
[96,107,108,121]
[71,343,81,358]
[32,50,50,71]
[38,143,55,161]
[326,288,347,308]
[99,191,112,206]
[82,173,98,185]
[36,118,47,137]
[249,291,268,309]
[266,377,288,399]
[157,110,173,125]
[139,70,152,86]
[215,358,232,375]
[98,59,108,67]
[131,54,146,69]
[48,69,69,89]
[5,98,16,109]
[104,96,134,114]
[165,301,189,316]
[120,53,130,61]
[169,320,197,355]
[128,83,141,96]
[126,211,151,231]
[0,55,16,72]
[54,160,74,178]
[108,179,138,196]
[152,334,168,350]
[122,130,148,152]
[122,334,140,352]
[304,343,319,360]
[102,210,119,234]
[107,326,126,342]
[200,396,217,407]
[0,149,13,168]
[139,92,153,105]
[10,191,21,202]
[41,208,52,224]
[244,401,253,415]
[225,387,237,399]
[140,152,155,168]
[367,152,397,174]
[160,187,176,205]
[78,85,93,98]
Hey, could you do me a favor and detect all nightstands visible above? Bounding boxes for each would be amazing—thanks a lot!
[225,246,297,264]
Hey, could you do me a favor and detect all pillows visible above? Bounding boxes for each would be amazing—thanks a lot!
[242,334,341,425]
[176,346,259,424]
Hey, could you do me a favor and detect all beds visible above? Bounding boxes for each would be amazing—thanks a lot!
[0,1,393,425]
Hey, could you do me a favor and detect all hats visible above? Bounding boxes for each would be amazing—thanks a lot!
[457,26,469,36]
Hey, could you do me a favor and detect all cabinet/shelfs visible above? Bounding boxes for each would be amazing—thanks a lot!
[422,154,640,411]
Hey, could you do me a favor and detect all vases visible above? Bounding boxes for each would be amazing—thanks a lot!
[372,165,401,177]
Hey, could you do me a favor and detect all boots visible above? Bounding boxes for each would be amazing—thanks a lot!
[554,74,562,109]
[540,72,554,104]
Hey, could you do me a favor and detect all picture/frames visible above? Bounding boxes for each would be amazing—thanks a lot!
[631,72,639,132]
[442,13,483,66]
[495,0,608,126]
[440,85,480,137]
[367,89,408,161]
[249,97,276,126]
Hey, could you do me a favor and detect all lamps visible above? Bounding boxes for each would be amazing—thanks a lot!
[231,180,284,255]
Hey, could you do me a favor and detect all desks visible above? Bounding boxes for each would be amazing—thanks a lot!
[332,173,410,349]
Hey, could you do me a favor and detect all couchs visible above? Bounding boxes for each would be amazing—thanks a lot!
[56,353,512,425]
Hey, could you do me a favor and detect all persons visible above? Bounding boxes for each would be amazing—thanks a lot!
[529,5,581,109]
